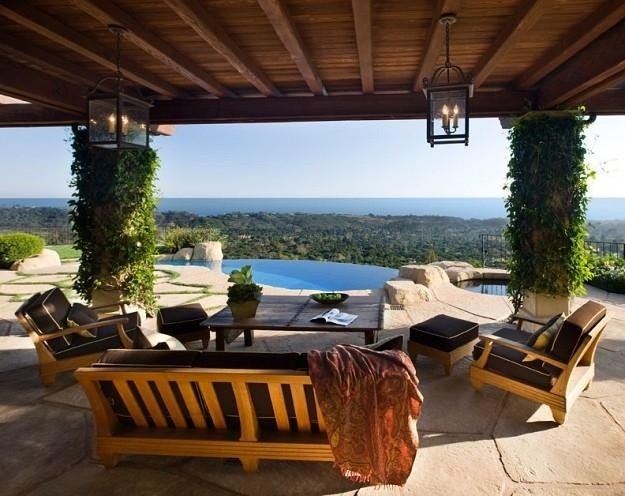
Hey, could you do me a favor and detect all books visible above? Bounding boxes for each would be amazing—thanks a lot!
[310,308,359,328]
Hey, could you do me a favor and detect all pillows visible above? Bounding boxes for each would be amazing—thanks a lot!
[521,312,564,362]
[66,303,98,339]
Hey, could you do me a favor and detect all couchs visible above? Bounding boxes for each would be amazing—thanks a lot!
[74,334,403,474]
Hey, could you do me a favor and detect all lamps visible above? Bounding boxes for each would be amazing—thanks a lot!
[83,26,154,151]
[422,16,473,147]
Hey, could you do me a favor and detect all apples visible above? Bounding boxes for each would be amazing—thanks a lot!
[314,293,342,300]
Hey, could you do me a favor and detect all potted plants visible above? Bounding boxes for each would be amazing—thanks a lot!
[226,266,263,318]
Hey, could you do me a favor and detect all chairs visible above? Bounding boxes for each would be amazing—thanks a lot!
[470,304,610,423]
[14,288,141,386]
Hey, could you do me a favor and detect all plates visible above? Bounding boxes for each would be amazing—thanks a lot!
[310,293,349,306]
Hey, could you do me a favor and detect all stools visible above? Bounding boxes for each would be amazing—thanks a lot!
[156,303,209,348]
[408,314,479,377]
